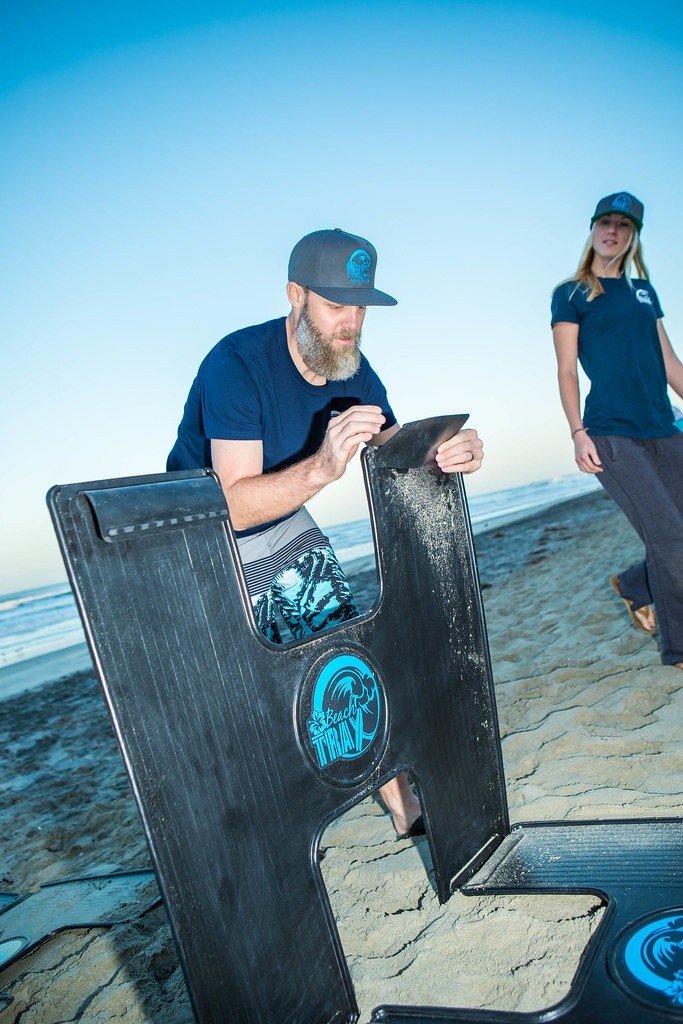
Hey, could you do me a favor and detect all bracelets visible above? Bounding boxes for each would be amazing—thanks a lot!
[572,428,583,439]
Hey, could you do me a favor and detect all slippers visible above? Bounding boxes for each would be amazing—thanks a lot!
[612,575,658,633]
[397,814,426,838]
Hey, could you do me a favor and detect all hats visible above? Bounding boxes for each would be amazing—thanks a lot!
[289,228,398,306]
[592,192,645,231]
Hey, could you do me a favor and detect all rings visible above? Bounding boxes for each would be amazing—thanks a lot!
[467,451,474,461]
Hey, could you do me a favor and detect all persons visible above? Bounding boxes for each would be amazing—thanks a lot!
[167,228,483,841]
[550,192,683,670]
